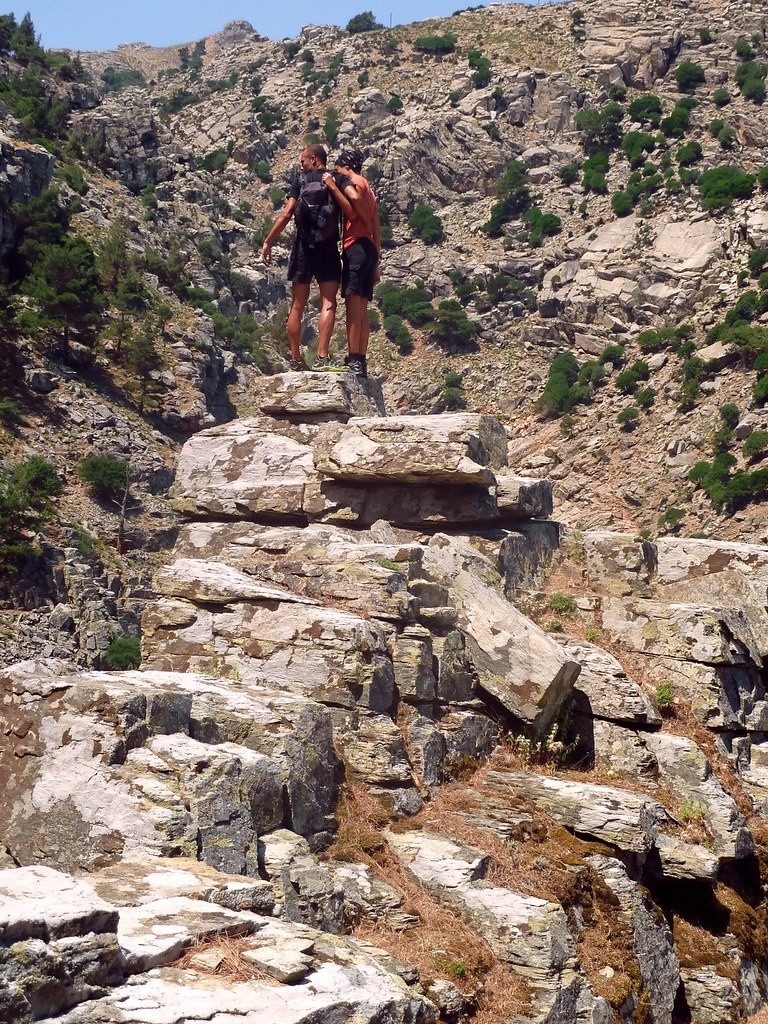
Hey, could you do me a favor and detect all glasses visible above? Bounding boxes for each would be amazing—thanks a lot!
[334,166,340,171]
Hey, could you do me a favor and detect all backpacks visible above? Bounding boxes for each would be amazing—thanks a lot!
[294,169,340,248]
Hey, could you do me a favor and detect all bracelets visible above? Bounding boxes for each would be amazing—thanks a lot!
[265,238,271,246]
[370,233,373,238]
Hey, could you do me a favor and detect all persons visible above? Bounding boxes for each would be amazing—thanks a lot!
[261,145,374,373]
[321,150,380,378]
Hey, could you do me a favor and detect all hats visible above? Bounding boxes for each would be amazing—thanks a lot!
[335,150,365,172]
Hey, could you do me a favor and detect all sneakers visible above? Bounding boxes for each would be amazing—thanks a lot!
[343,359,363,374]
[313,353,341,367]
[290,359,311,370]
[360,363,368,377]
